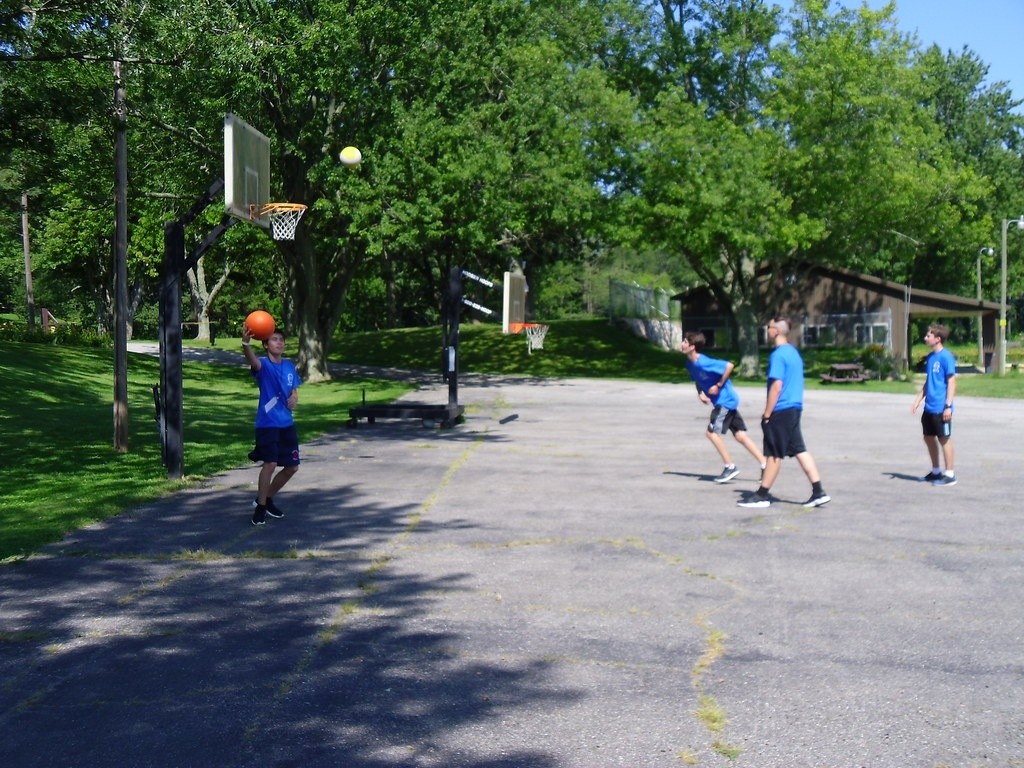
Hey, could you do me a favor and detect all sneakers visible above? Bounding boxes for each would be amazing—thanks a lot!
[933,475,958,487]
[252,496,284,519]
[759,468,765,482]
[714,465,739,482]
[920,472,943,481]
[802,489,831,508]
[737,492,771,507]
[251,504,267,525]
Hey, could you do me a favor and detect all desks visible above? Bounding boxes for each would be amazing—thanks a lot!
[828,363,860,384]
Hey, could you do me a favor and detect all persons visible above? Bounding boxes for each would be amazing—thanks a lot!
[911,323,958,486]
[735,316,831,508]
[241,320,300,527]
[681,331,766,484]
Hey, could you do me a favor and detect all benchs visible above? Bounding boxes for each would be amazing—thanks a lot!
[816,373,870,384]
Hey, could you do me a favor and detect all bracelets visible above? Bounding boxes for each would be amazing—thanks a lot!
[944,403,951,409]
[715,382,721,389]
[760,414,770,420]
[241,341,250,346]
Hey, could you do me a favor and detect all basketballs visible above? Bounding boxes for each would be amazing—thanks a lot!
[245,309,275,341]
[340,146,362,168]
